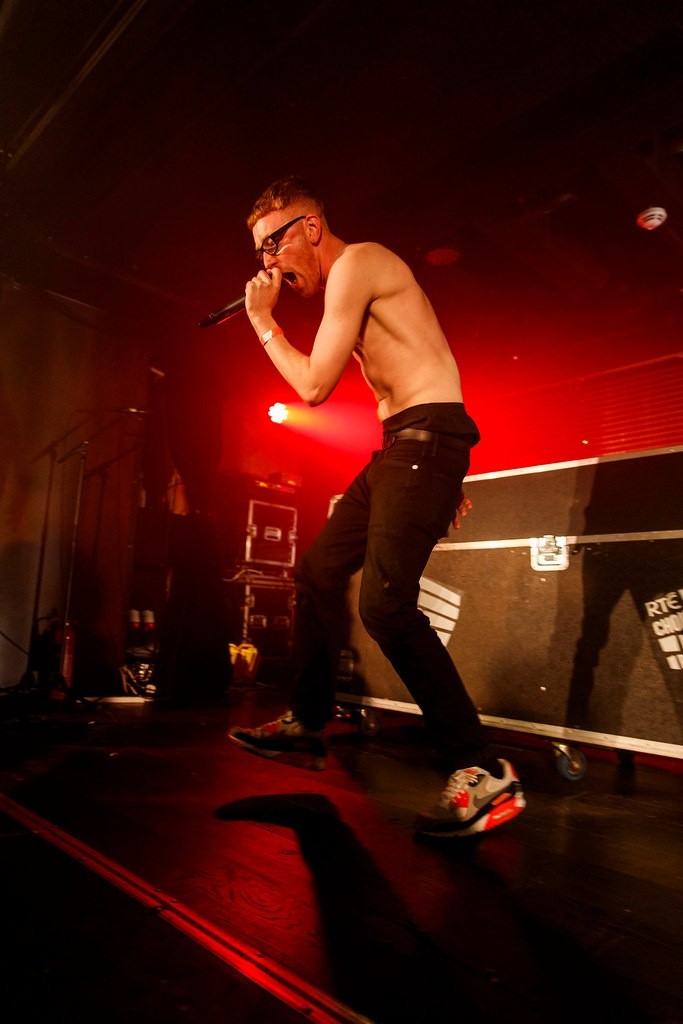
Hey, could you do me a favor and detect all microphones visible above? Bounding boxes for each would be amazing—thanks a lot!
[198,275,273,327]
[107,407,146,416]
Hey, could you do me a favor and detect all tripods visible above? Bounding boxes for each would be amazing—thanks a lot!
[0,412,126,712]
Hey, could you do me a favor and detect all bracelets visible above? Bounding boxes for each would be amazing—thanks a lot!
[258,327,284,347]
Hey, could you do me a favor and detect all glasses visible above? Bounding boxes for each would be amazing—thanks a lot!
[254,215,307,260]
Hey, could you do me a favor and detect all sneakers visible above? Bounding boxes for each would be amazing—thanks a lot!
[228,715,326,770]
[421,758,525,835]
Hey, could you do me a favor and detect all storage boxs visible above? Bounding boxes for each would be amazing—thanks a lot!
[327,445,683,789]
[245,499,298,568]
[238,583,297,662]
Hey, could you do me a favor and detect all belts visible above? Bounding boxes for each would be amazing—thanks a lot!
[381,427,468,452]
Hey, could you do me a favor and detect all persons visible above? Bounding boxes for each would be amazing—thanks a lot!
[227,174,525,836]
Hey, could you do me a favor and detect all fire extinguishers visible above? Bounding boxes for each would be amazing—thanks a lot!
[38,609,76,702]
